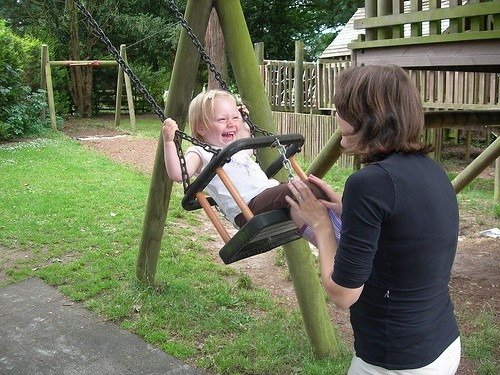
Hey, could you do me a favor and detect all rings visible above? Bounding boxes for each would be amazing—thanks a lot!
[296,195,303,201]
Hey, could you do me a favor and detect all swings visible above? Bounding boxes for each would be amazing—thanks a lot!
[74,0,309,265]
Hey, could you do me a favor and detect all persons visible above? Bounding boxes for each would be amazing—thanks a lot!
[283,62,462,375]
[161,88,344,258]
